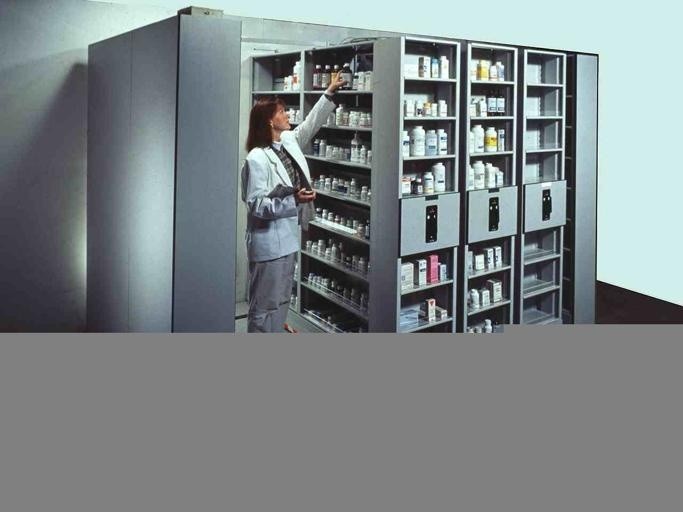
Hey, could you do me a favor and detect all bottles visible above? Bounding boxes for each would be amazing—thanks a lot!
[284,54,505,333]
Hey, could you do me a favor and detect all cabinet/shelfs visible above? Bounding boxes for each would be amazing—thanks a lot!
[246,35,405,334]
[566,53,596,327]
[520,47,564,327]
[402,39,461,331]
[464,42,517,331]
[84,15,243,333]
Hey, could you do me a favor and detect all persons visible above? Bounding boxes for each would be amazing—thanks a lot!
[240,66,351,331]
